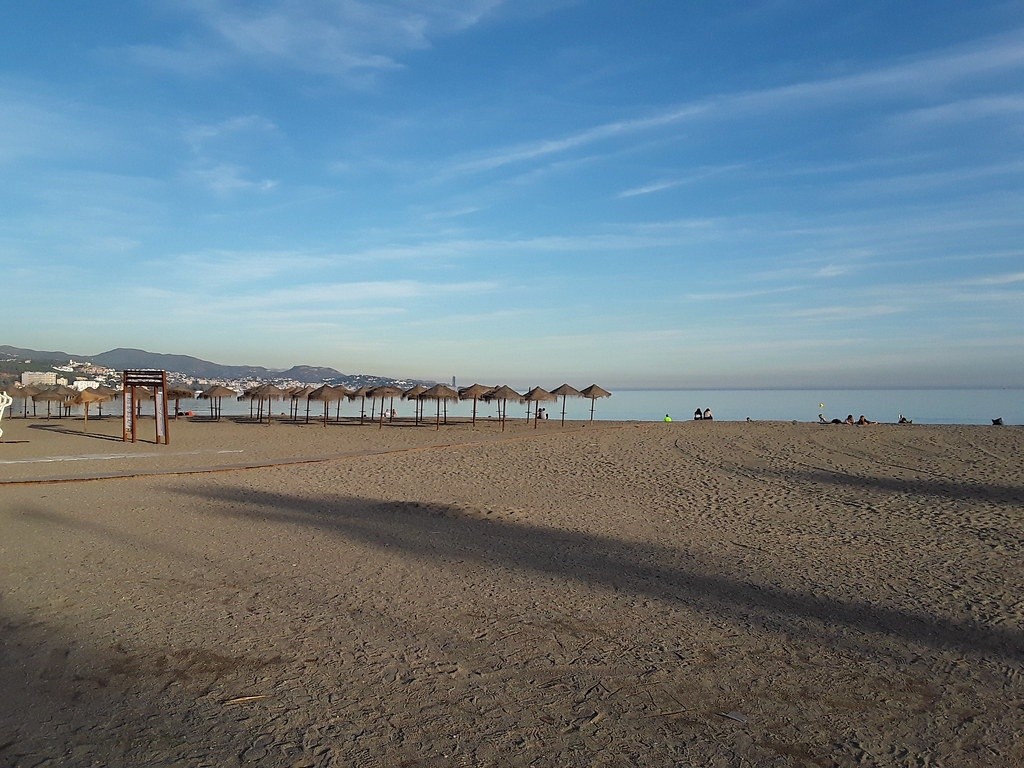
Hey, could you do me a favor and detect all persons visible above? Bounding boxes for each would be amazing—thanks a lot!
[664,414,671,421]
[818,414,872,426]
[899,414,912,423]
[537,408,542,419]
[694,408,713,420]
[541,408,548,419]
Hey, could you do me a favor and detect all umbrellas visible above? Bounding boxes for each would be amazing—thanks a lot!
[581,384,610,421]
[550,384,580,427]
[335,383,404,429]
[0,382,117,425]
[118,387,150,418]
[237,384,287,426]
[403,383,458,431]
[458,384,522,431]
[167,389,193,419]
[197,385,236,419]
[285,384,343,426]
[522,386,556,428]
